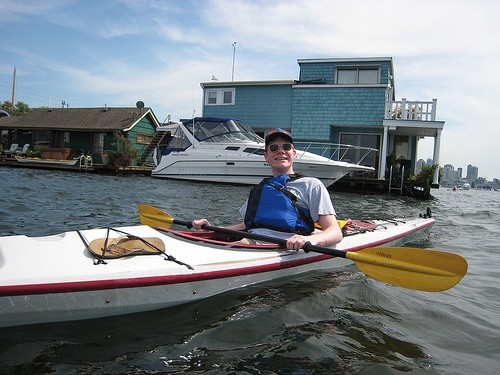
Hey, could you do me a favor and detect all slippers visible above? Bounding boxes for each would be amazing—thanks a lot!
[88,235,166,258]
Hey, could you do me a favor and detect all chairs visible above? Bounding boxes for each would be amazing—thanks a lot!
[18,144,30,158]
[4,144,17,157]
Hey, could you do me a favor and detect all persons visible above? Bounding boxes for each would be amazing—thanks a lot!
[193,128,342,252]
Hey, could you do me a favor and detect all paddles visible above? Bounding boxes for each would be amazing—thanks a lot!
[137,202,470,293]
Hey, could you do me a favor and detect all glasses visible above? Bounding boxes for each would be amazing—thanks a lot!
[268,143,295,153]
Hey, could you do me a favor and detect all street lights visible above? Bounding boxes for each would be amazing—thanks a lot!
[231,41,238,82]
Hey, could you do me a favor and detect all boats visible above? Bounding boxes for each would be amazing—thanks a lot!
[150,114,378,193]
[0,207,437,330]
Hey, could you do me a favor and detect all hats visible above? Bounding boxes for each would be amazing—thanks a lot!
[264,127,294,152]
